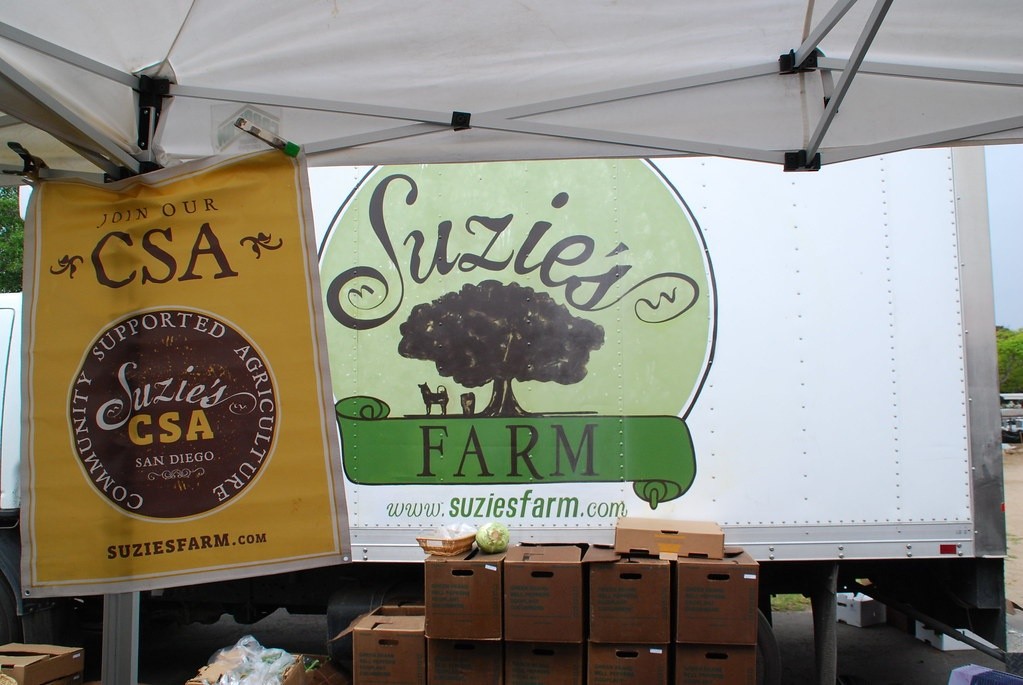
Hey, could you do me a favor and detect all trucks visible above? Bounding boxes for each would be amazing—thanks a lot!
[999,393,1023,435]
[0,140,1008,658]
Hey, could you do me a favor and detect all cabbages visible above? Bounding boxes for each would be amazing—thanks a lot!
[475,522,510,553]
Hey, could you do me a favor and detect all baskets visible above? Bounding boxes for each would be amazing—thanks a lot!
[416,534,476,557]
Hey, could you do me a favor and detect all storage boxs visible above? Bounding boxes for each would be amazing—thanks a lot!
[327,541,759,685]
[836,593,887,627]
[186,649,350,685]
[0,643,85,685]
[615,517,725,559]
[915,599,1023,651]
